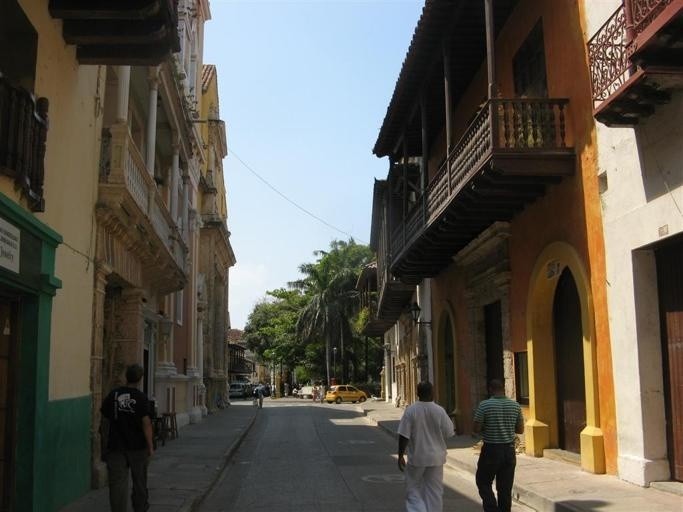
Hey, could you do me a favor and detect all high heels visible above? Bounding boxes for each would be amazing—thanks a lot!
[98,421,118,461]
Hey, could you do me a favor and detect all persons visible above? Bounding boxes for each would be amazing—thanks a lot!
[250,381,327,406]
[99,363,155,512]
[398,380,453,512]
[471,378,524,512]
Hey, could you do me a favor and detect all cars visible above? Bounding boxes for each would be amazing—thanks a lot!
[325,384,367,404]
[229,383,246,398]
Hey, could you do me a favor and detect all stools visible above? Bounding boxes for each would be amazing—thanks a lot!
[161,412,177,441]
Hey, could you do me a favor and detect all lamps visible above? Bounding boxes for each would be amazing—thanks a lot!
[408,299,431,327]
